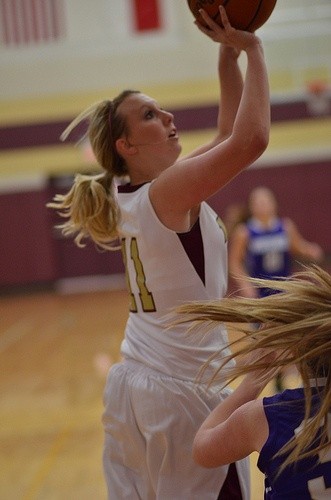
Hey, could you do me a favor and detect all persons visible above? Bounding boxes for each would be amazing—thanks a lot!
[159,262,331,500]
[45,5,271,500]
[228,184,325,394]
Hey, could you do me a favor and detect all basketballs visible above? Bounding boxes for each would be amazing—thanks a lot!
[186,1,277,34]
